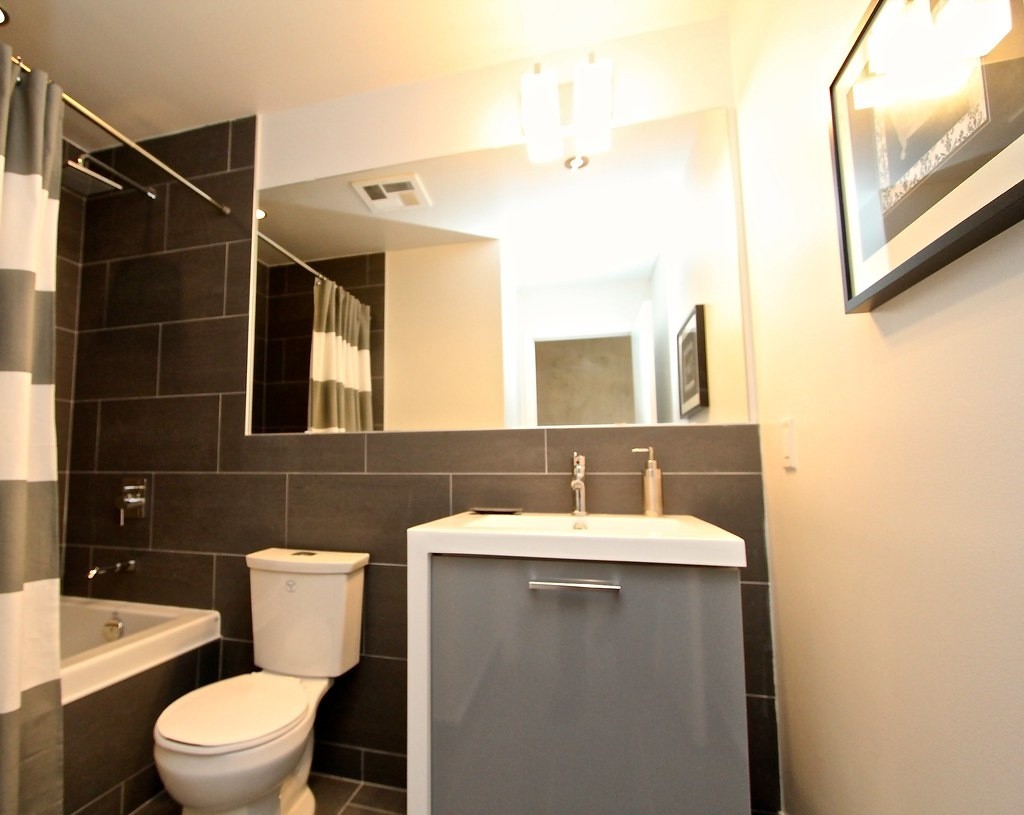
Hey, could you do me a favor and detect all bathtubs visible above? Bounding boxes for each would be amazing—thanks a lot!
[59,593,222,814]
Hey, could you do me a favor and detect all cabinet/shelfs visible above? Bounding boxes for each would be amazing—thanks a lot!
[430,556,750,813]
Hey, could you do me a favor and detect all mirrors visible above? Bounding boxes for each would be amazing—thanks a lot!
[252,106,750,434]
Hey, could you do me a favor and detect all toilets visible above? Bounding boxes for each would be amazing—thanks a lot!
[153,546,369,815]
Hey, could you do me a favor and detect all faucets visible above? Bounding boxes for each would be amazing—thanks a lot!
[570,451,588,516]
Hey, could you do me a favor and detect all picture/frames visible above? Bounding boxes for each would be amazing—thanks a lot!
[676,306,708,419]
[830,0,1024,313]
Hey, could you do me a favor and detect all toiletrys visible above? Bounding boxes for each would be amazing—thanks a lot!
[631,446,664,517]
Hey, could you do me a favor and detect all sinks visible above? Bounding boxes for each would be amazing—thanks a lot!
[460,513,695,539]
